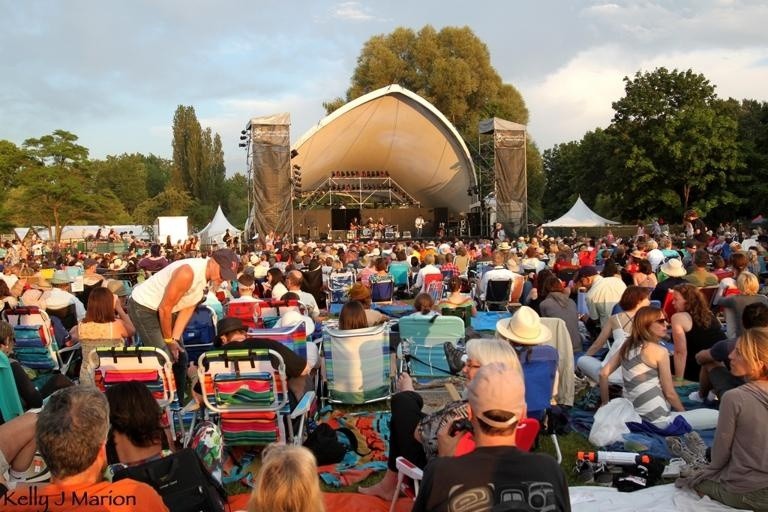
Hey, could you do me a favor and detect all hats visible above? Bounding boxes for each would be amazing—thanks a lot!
[496,307,552,345]
[469,363,525,428]
[214,248,239,281]
[213,318,248,350]
[20,269,130,305]
[660,259,687,277]
[499,240,510,251]
[630,250,647,258]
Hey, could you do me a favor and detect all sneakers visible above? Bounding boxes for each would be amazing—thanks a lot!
[594,464,613,487]
[666,432,709,467]
[444,342,466,371]
[571,460,595,484]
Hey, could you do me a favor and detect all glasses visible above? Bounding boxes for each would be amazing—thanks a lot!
[654,318,667,327]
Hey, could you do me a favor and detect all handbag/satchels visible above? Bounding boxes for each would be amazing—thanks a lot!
[113,448,228,512]
[303,423,344,466]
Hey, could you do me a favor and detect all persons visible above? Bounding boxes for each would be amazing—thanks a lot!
[0,214,768,512]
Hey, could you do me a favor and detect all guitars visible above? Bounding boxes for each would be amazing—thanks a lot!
[416,221,429,228]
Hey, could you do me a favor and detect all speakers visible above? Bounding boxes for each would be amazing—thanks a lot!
[434,207,448,236]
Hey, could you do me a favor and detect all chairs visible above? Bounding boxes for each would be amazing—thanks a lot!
[0,253,768,512]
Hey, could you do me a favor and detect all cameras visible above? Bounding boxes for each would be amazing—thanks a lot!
[450,418,474,437]
